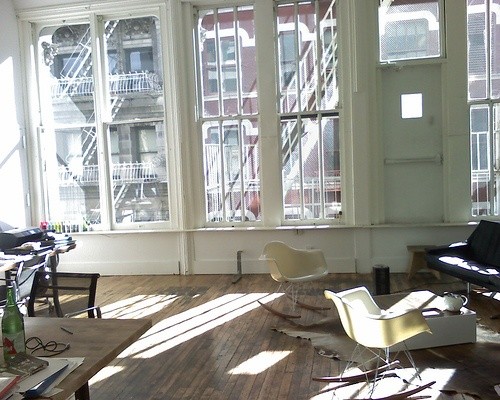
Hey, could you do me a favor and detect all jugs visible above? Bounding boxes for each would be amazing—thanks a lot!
[444,294,468,311]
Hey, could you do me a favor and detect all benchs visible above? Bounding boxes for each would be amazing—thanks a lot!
[424,219,500,319]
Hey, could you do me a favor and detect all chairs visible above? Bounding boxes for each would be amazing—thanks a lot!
[255,241,328,318]
[324,286,436,400]
[27,272,102,318]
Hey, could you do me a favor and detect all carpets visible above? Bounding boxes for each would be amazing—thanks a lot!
[273,293,500,400]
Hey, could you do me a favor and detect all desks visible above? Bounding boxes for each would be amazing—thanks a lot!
[405,245,450,282]
[0,318,152,400]
[374,290,477,352]
[0,244,76,317]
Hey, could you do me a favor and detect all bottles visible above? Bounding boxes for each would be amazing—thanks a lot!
[1,286,27,367]
